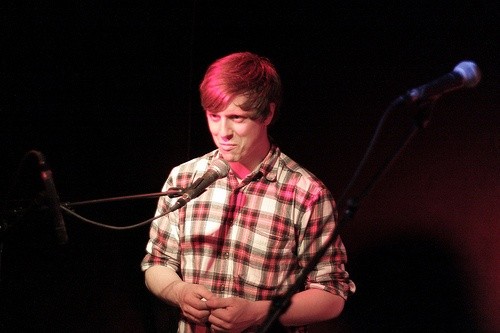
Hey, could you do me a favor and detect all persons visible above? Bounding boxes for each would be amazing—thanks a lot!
[141,51,356,333]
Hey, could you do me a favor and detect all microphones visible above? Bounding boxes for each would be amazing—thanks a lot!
[25,149,70,247]
[393,59,482,109]
[174,158,229,211]
